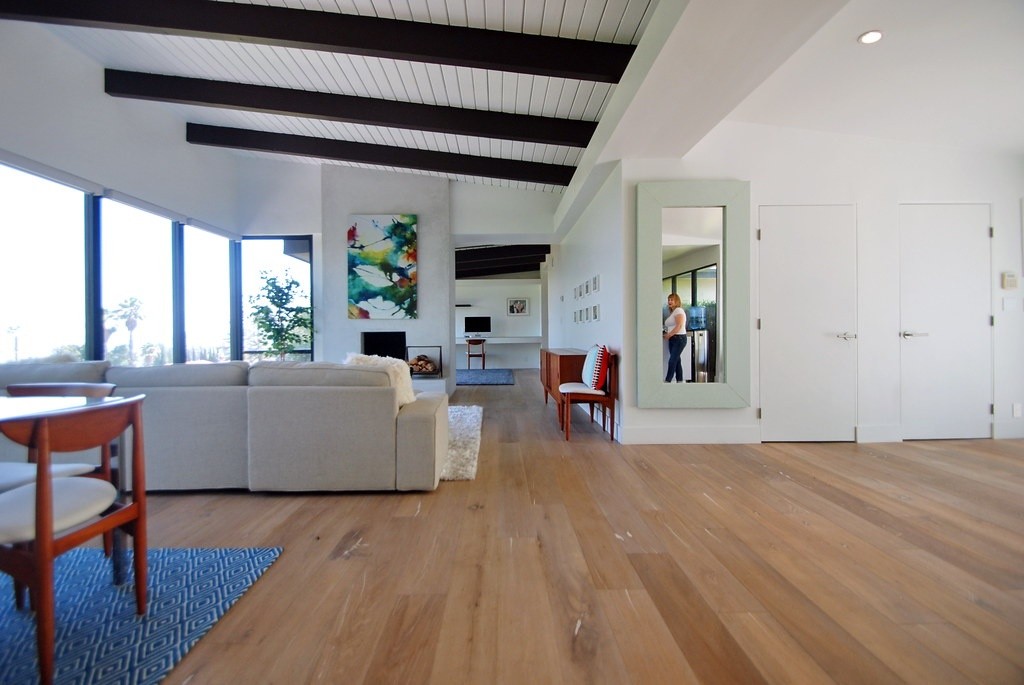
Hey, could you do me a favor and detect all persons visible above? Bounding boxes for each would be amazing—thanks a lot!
[664,293,687,382]
[510,301,521,313]
[518,304,526,313]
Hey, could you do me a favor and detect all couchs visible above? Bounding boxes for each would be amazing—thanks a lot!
[0,356,449,491]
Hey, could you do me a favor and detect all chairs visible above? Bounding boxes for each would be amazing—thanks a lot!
[0,383,147,685]
[560,352,617,441]
[465,339,487,370]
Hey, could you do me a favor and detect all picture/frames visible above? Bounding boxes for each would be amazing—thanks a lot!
[507,298,530,316]
[573,274,599,300]
[573,304,600,323]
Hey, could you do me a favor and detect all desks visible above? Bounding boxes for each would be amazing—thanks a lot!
[663,332,693,383]
[1,396,123,421]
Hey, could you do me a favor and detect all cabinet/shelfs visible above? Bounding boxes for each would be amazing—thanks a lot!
[539,348,593,425]
[411,376,449,396]
[455,368,515,385]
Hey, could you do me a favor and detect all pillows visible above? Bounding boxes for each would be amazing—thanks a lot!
[582,344,609,390]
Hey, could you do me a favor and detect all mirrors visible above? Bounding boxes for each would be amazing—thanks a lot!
[637,179,751,409]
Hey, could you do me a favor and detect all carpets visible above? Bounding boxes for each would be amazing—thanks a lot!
[0,547,284,685]
[440,405,484,479]
